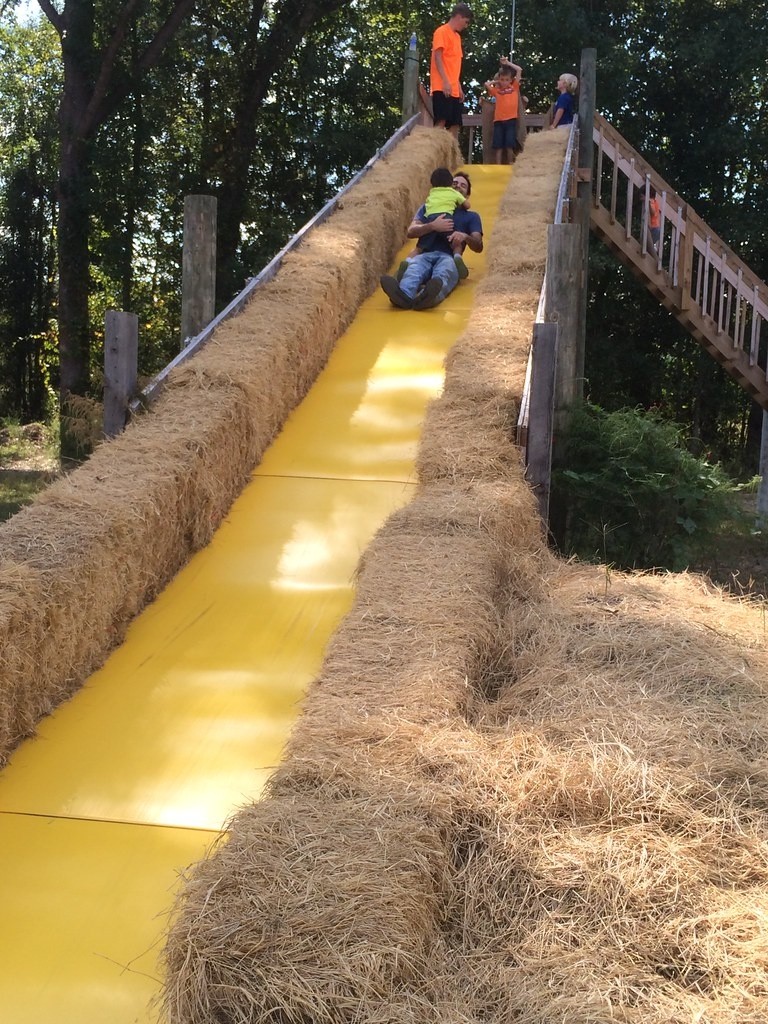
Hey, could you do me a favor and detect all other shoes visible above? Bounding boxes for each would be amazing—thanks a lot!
[412,275,445,312]
[454,252,469,280]
[379,274,415,310]
[396,259,410,285]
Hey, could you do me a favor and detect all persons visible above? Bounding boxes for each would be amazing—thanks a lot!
[549,73,577,130]
[380,171,483,310]
[397,168,470,282]
[430,2,472,139]
[639,184,660,245]
[484,55,521,164]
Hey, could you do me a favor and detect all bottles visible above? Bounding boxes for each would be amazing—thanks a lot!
[409,32,417,52]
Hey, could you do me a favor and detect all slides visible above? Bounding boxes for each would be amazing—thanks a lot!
[1,162,514,1024]
